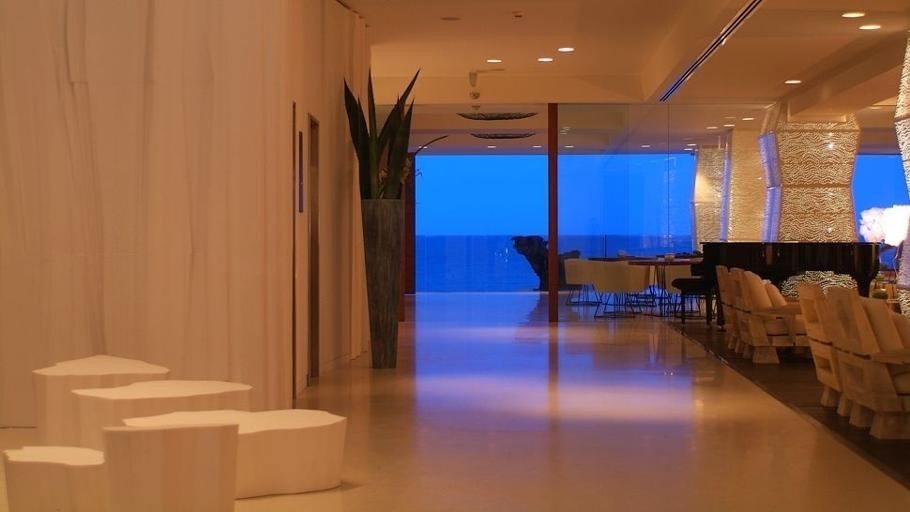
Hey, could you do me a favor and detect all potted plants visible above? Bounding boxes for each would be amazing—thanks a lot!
[343,62,447,370]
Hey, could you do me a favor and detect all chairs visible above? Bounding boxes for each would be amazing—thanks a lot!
[796,280,909,439]
[564,256,710,321]
[715,266,812,364]
